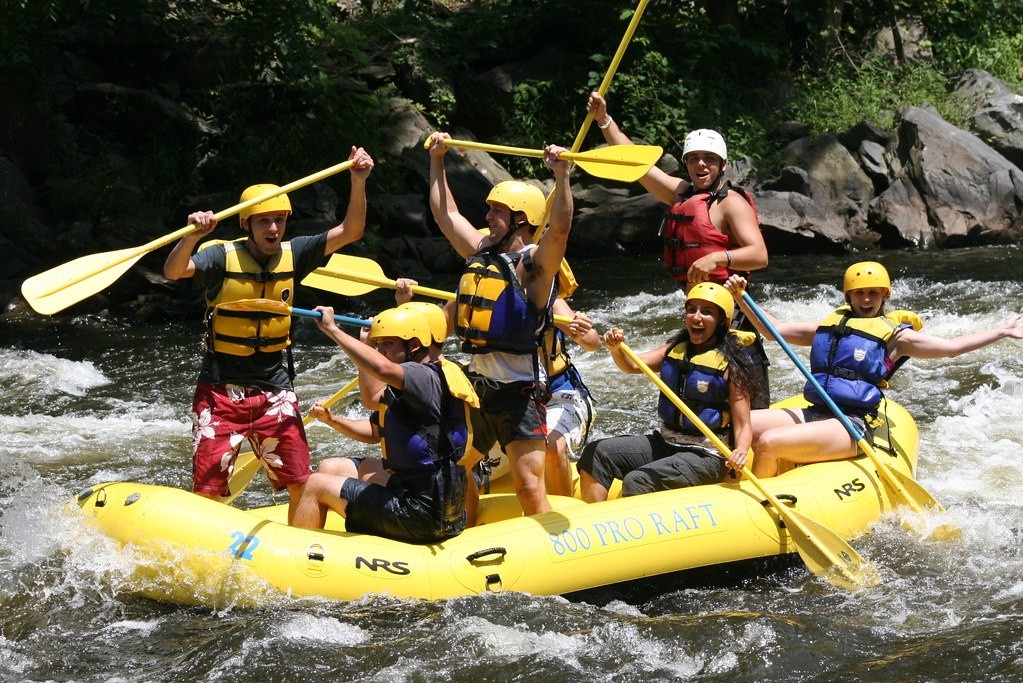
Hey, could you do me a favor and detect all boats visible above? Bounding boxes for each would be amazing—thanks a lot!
[49,388,920,609]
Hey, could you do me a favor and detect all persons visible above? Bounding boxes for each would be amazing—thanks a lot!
[723,262,1023,479]
[586,92,770,410]
[290,302,481,543]
[395,133,603,529]
[576,282,764,504]
[164,147,374,527]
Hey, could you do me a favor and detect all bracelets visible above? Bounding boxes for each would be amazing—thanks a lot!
[597,115,612,129]
[725,251,731,268]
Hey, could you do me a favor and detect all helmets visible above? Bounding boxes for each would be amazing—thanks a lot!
[684,284,735,330]
[843,263,892,303]
[239,183,292,228]
[486,179,547,228]
[680,128,727,165]
[400,302,447,343]
[369,308,432,347]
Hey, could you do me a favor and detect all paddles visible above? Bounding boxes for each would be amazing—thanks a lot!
[19,157,366,318]
[221,376,360,506]
[423,130,665,184]
[533,0,649,246]
[289,305,373,329]
[606,328,882,590]
[298,251,584,335]
[728,281,964,542]
